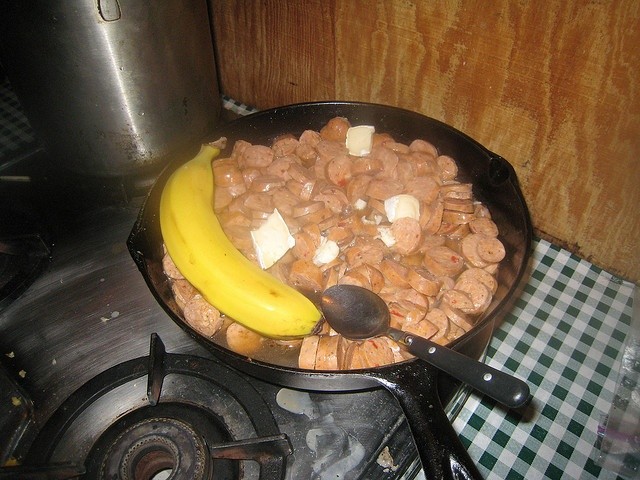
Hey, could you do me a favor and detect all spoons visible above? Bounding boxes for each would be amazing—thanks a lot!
[320,284,530,411]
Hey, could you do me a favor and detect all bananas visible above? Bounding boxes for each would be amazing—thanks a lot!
[159,136,326,341]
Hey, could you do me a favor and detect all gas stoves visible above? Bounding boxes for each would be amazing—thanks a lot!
[0,106,496,480]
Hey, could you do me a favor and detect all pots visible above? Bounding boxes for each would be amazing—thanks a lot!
[0,1,221,179]
[126,100,533,480]
